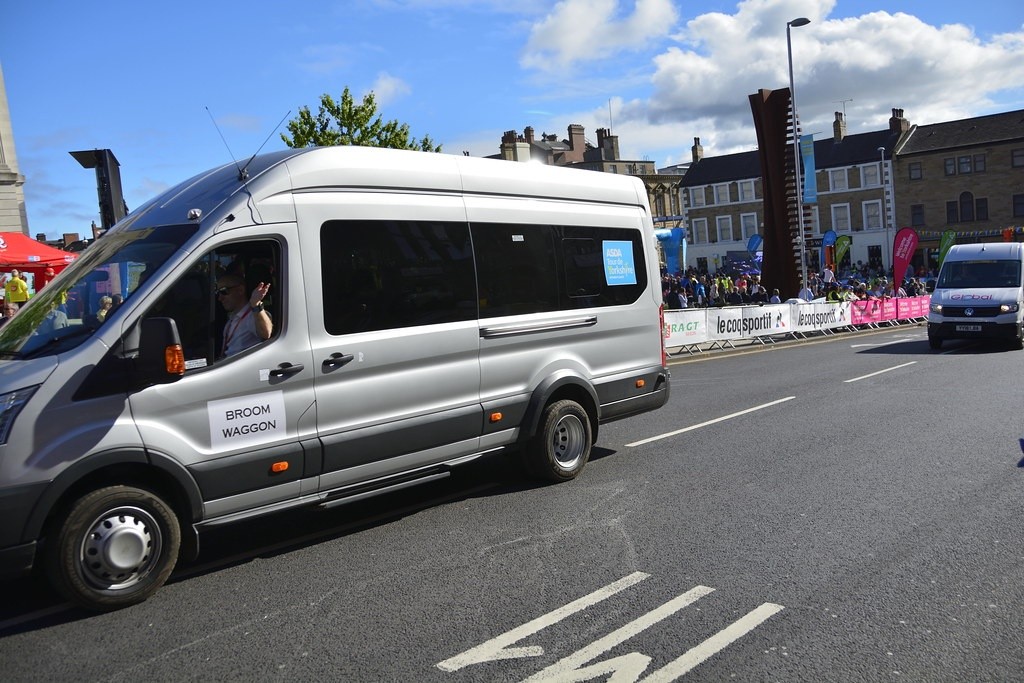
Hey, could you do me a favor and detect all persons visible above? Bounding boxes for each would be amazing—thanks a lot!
[18,270,27,282]
[0,303,20,327]
[103,293,124,321]
[5,269,30,309]
[214,273,274,359]
[43,267,59,286]
[659,256,940,311]
[35,300,70,335]
[96,295,112,323]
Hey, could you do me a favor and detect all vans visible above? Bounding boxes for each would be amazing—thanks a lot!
[1,143,671,612]
[924,240,1023,348]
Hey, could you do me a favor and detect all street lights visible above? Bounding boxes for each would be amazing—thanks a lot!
[783,14,811,295]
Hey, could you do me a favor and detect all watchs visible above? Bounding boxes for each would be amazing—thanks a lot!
[253,302,266,312]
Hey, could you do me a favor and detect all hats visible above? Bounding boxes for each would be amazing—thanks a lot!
[45,268,54,273]
[832,282,840,287]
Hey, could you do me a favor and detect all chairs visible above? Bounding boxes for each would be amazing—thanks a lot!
[245,267,274,304]
[167,275,213,353]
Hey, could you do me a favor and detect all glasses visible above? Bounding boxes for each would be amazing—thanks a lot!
[3,307,12,310]
[213,282,238,296]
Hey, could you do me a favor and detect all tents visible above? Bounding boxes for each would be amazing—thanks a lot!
[0,231,81,314]
